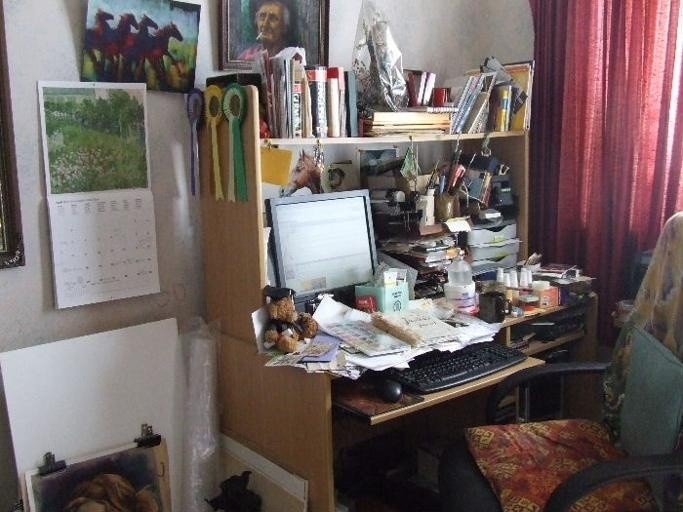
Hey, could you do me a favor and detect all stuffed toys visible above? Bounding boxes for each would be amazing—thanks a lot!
[262,284,319,354]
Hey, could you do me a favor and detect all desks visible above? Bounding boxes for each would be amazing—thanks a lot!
[194,66,608,511]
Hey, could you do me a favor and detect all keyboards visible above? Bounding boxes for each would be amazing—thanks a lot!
[383,340,528,393]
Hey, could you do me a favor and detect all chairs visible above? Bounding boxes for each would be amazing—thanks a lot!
[434,211,683,510]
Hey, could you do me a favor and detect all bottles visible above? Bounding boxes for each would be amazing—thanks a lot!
[447,247,471,285]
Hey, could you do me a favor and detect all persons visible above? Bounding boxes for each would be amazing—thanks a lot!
[236,0,304,62]
[63,468,160,512]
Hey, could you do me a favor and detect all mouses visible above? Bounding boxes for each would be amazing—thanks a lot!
[379,378,403,400]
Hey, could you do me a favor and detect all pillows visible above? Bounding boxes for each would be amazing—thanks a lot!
[617,325,682,510]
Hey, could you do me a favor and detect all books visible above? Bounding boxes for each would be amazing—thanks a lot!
[257,48,519,139]
[374,307,459,347]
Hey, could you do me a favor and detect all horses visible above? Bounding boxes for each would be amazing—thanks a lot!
[84,8,184,81]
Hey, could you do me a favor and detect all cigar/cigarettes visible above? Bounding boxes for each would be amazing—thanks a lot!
[256,32,262,41]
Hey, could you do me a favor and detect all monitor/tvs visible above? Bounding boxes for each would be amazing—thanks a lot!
[266,188,378,298]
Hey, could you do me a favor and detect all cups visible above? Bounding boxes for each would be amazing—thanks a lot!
[443,280,477,315]
[496,266,531,288]
[433,193,453,222]
[432,86,451,108]
[478,290,511,324]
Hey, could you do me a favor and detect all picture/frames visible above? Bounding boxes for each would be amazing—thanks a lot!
[217,1,331,76]
[0,2,27,271]
